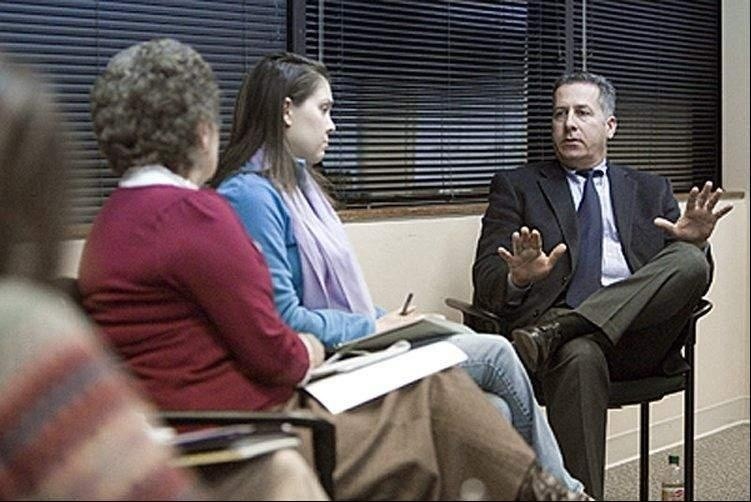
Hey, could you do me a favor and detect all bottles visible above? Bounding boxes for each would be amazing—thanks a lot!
[661,452,688,502]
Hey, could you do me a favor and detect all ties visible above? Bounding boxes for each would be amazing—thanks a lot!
[566,170,604,308]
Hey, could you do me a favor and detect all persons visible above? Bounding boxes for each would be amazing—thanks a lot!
[473,71,733,501]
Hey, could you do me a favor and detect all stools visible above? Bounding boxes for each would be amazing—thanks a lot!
[603,292,714,498]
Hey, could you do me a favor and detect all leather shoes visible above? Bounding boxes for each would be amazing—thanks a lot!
[512,323,560,371]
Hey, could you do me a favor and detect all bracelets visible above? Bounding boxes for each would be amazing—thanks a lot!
[507,272,532,293]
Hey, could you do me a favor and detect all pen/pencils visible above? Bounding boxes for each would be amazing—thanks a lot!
[401,294,412,315]
